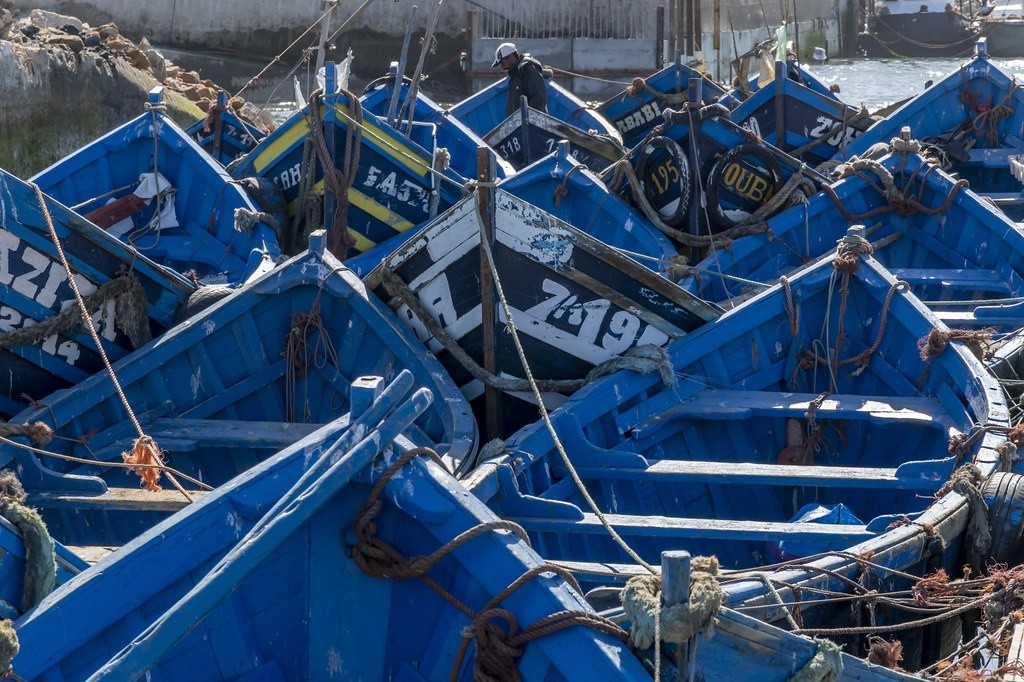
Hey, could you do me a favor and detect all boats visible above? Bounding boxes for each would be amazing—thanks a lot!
[0,0,1024,680]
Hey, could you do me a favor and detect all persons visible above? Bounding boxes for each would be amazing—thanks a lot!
[492,42,546,117]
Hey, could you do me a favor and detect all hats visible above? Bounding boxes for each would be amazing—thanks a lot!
[491,42,517,67]
